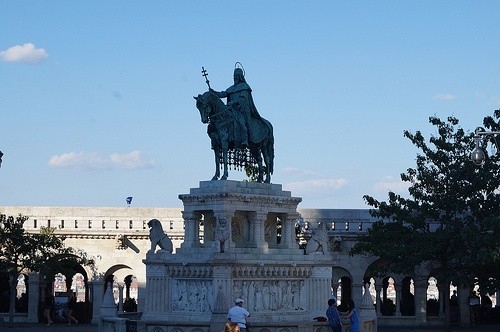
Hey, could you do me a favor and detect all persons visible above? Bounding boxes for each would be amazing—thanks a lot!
[43,290,54,326]
[116,297,136,312]
[209,67,252,146]
[224,320,241,331]
[227,298,251,331]
[340,299,360,331]
[65,289,79,326]
[326,298,344,331]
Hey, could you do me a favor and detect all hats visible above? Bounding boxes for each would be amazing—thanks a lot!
[235,299,244,304]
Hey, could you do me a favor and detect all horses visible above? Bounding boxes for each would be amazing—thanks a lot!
[192,92,274,183]
